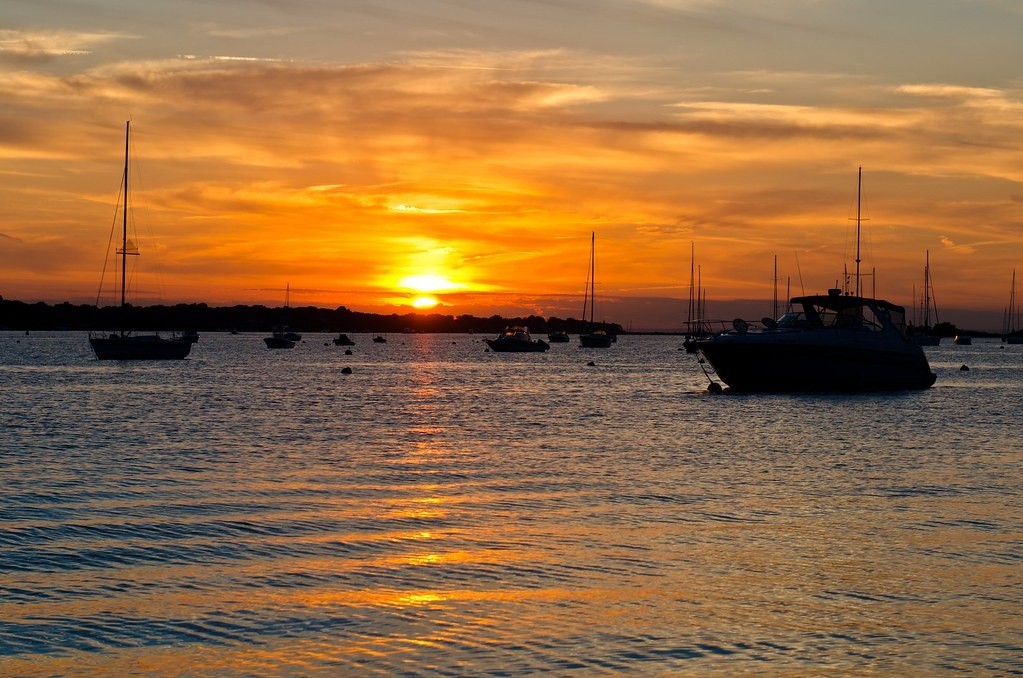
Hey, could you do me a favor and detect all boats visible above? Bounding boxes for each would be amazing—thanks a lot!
[181,329,200,343]
[373,336,387,343]
[692,288,938,394]
[547,330,570,342]
[332,333,356,347]
[482,324,552,352]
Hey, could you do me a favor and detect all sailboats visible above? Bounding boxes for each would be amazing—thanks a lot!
[88,117,200,360]
[262,281,302,348]
[684,169,1023,359]
[578,230,618,349]
